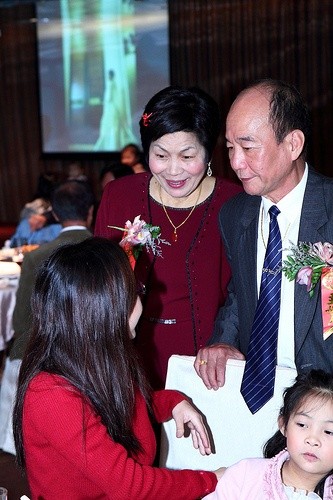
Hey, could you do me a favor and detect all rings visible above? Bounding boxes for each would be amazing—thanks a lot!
[200,360,207,364]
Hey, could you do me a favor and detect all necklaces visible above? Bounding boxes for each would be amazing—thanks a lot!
[159,182,202,242]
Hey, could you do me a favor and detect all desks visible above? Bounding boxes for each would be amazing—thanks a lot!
[0,260,23,351]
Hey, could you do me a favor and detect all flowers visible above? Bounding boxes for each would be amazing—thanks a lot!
[106,215,170,261]
[260,240,333,299]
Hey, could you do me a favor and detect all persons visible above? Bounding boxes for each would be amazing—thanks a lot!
[93,86,243,389]
[8,162,89,256]
[11,179,94,359]
[13,237,229,500]
[201,369,333,500]
[193,78,333,414]
[100,143,146,186]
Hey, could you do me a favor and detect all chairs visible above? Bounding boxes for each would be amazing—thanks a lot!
[160,354,296,472]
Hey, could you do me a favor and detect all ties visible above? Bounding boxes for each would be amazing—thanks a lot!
[239,205,281,414]
